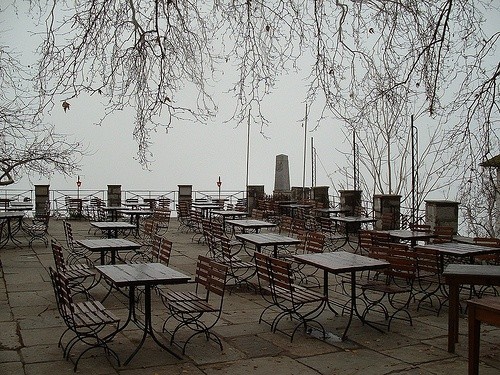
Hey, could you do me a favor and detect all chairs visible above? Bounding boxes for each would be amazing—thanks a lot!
[0,196,500,373]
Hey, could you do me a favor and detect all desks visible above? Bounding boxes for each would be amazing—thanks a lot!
[210,211,249,240]
[76,238,142,289]
[443,263,500,352]
[194,205,223,234]
[235,233,303,290]
[0,211,25,249]
[102,207,129,233]
[330,216,377,254]
[412,242,500,315]
[464,296,500,375]
[274,201,298,225]
[95,263,191,366]
[91,222,137,265]
[117,210,153,239]
[280,205,314,230]
[292,250,391,340]
[371,230,450,283]
[313,208,350,235]
[225,220,277,254]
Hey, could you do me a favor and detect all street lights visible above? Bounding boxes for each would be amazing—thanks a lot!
[217,176,222,199]
[77,175,82,198]
